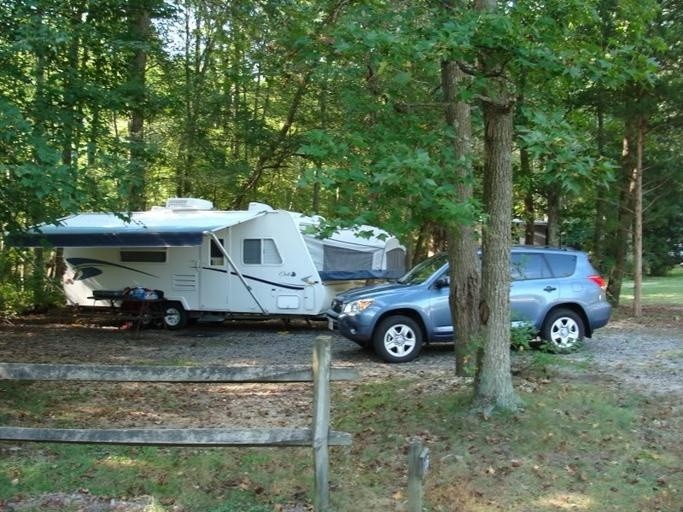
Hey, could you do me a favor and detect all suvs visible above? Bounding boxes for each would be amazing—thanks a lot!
[324,245,614,364]
